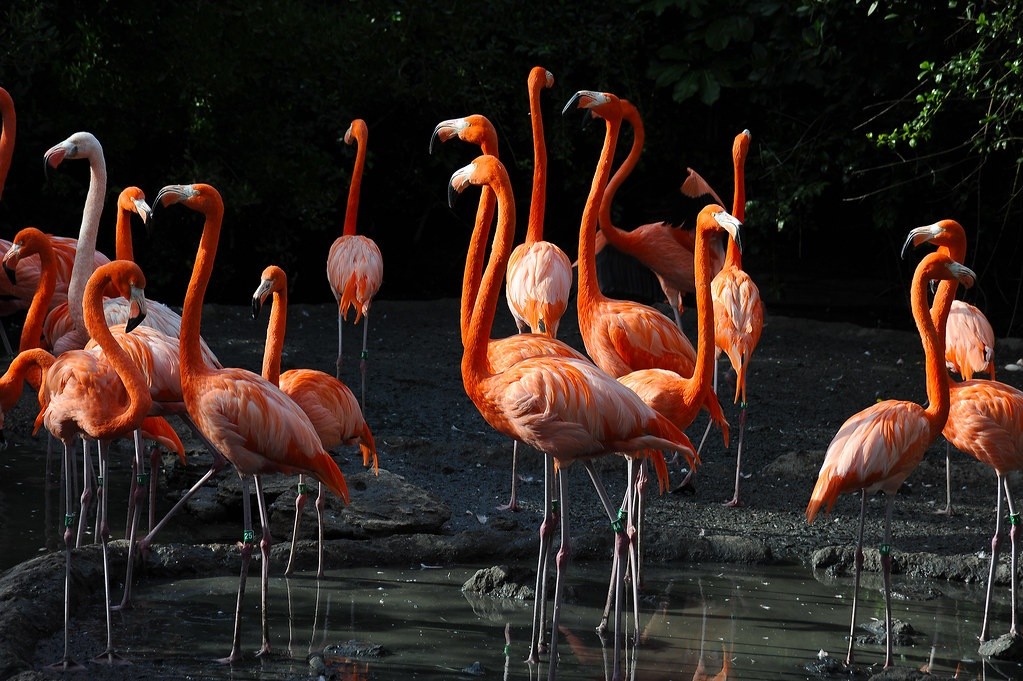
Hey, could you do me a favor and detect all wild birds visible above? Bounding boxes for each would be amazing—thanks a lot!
[429,65,766,681]
[1,81,386,669]
[800,216,1022,681]
